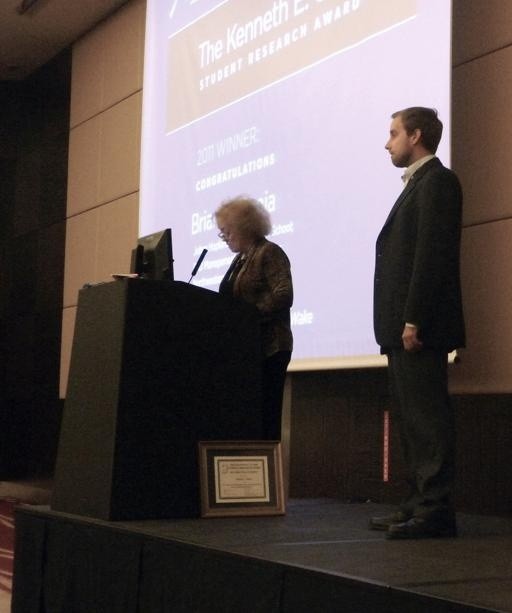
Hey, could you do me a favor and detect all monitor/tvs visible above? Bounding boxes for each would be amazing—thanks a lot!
[130,227,175,281]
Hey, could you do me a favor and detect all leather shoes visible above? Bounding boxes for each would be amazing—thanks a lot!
[386,516,457,539]
[370,511,409,530]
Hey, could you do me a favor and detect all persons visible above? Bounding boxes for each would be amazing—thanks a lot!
[211,196,293,442]
[368,107,467,540]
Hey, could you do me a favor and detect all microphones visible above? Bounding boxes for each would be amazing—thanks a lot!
[191,249,207,277]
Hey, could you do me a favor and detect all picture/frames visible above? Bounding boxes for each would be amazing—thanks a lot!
[197,439,287,518]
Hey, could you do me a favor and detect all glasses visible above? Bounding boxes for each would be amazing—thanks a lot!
[218,231,235,241]
